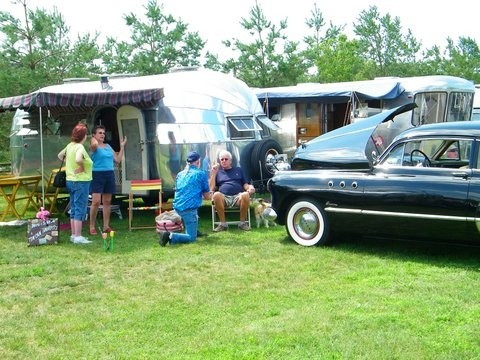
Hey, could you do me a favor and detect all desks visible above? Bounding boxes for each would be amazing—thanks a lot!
[0,176,43,222]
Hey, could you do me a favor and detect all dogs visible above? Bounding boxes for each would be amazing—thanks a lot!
[254,200,277,229]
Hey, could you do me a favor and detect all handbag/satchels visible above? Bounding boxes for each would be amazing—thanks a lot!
[53,171,66,187]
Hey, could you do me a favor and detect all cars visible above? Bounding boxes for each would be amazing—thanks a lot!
[262,103,480,249]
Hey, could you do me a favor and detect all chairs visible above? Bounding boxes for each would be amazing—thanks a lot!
[130,179,163,233]
[209,190,249,231]
[0,169,64,220]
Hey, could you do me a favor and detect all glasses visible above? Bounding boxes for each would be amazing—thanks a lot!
[447,149,455,152]
[221,158,228,161]
[95,131,105,134]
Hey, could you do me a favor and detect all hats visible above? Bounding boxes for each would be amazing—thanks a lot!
[447,145,458,150]
[186,151,200,162]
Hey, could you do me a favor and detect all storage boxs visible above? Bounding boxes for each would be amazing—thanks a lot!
[27,219,59,245]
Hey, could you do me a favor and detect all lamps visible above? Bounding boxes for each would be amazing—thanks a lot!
[101,77,110,90]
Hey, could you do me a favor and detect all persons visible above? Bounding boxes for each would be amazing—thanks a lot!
[159,151,210,247]
[58,123,93,244]
[90,125,127,235]
[211,150,255,232]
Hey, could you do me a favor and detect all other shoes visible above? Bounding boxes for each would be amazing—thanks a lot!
[213,224,228,232]
[238,221,252,230]
[70,235,88,242]
[159,232,170,246]
[74,236,92,243]
